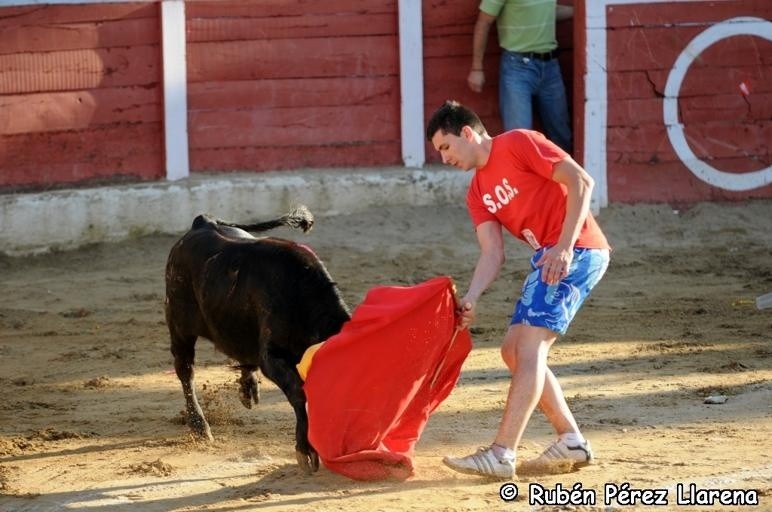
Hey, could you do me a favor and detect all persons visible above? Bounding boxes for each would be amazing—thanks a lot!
[426,99,613,480]
[467,2,573,156]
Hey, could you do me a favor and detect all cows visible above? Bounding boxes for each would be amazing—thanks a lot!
[162,201,356,478]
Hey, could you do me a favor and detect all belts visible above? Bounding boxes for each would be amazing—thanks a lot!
[509,49,560,62]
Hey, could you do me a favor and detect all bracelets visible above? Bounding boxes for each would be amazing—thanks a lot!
[471,68,485,72]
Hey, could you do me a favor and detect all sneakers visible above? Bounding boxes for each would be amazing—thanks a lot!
[443,448,516,478]
[524,437,592,468]
